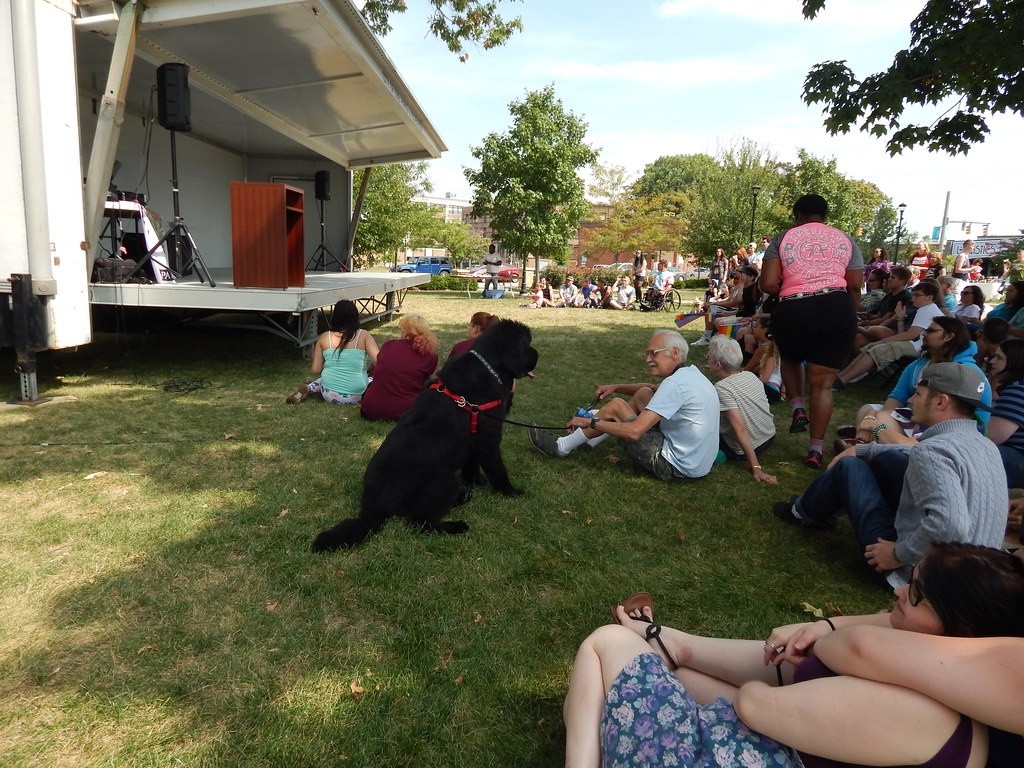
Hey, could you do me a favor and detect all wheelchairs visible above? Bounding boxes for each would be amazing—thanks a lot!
[640,284,681,313]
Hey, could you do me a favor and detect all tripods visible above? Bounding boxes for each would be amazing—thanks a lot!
[304,201,352,273]
[121,130,216,287]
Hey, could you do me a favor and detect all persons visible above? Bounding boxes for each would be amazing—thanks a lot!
[361,315,439,422]
[708,333,778,485]
[528,250,674,310]
[691,237,785,405]
[760,194,865,467]
[449,312,516,413]
[774,239,1024,594]
[483,244,502,290]
[562,544,1024,768]
[286,300,379,405]
[529,331,720,483]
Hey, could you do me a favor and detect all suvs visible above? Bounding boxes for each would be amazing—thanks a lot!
[388,255,456,280]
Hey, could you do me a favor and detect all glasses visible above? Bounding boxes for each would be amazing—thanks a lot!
[910,293,927,298]
[925,328,948,333]
[908,564,932,607]
[961,291,974,296]
[646,348,669,358]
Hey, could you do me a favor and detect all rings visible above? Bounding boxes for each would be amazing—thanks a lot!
[766,641,775,649]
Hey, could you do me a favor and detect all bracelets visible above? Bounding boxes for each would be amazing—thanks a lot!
[873,424,888,442]
[864,416,877,420]
[822,618,835,631]
[893,548,902,564]
[753,465,761,469]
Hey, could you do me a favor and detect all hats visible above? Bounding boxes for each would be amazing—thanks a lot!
[748,242,757,249]
[918,362,992,413]
[739,266,759,279]
[567,276,574,281]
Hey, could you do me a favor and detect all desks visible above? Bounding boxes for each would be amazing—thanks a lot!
[450,275,525,298]
[230,182,305,290]
[97,201,177,282]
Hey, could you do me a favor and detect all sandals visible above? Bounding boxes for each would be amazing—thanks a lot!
[611,592,678,670]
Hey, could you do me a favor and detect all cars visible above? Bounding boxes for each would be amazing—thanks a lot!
[650,266,688,282]
[533,264,567,275]
[590,262,651,278]
[689,267,712,279]
[469,263,523,283]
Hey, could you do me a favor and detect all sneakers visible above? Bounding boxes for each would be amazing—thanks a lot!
[577,442,592,452]
[528,423,570,459]
[806,450,823,468]
[789,408,809,433]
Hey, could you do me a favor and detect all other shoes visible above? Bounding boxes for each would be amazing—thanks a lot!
[831,378,845,391]
[790,494,835,531]
[834,438,867,453]
[837,425,856,438]
[773,502,812,528]
[286,385,310,406]
[690,335,710,345]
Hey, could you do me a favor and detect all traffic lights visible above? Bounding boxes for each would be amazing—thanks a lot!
[966,226,971,234]
[983,227,988,236]
[857,227,864,237]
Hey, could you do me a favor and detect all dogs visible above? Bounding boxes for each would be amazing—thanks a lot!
[684,297,702,315]
[309,321,539,555]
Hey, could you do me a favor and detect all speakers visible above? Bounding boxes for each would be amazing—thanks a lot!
[315,171,331,200]
[156,63,192,131]
[167,233,193,276]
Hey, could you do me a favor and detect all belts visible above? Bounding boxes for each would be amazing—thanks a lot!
[779,286,847,302]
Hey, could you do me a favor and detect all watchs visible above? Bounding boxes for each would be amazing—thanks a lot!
[590,416,600,429]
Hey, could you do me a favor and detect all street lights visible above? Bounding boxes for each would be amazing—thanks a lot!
[892,201,907,266]
[747,183,761,243]
